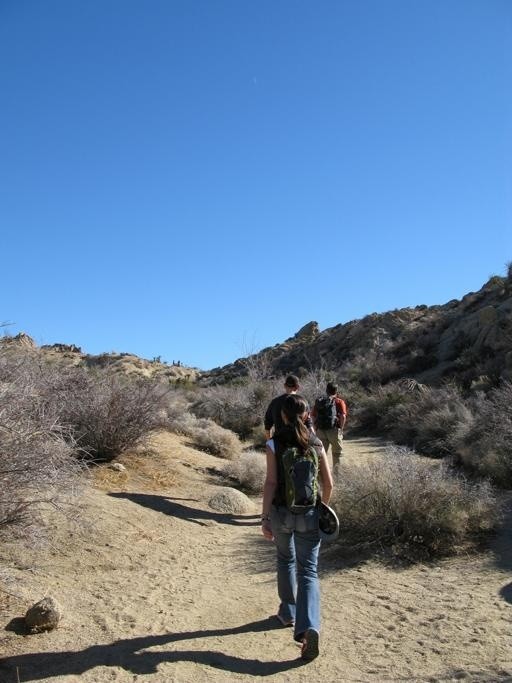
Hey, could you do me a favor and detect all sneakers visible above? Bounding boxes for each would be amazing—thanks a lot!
[277,614,296,627]
[301,628,318,661]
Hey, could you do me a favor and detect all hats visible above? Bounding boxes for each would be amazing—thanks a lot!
[318,501,340,543]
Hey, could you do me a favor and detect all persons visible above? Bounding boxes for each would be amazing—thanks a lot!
[264,373,317,439]
[310,380,348,472]
[260,394,334,660]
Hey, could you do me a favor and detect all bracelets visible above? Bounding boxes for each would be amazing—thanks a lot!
[261,510,272,522]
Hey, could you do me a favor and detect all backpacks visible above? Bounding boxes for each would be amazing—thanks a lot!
[283,433,319,514]
[315,396,337,429]
